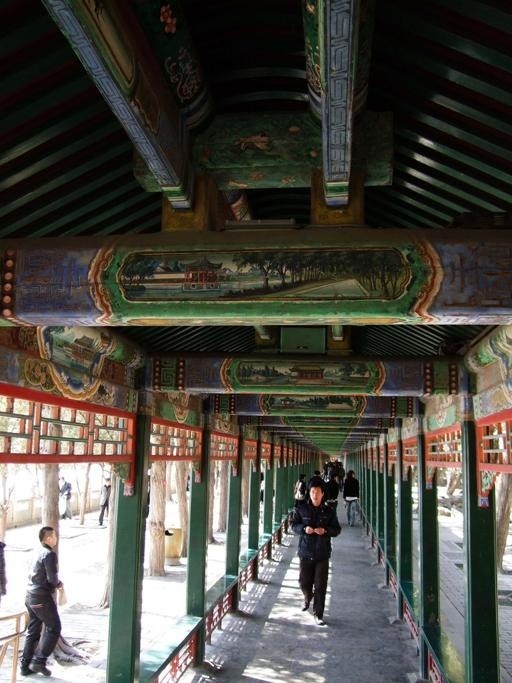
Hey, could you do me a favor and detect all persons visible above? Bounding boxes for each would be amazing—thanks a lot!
[324,473,339,504]
[98,477,111,526]
[17,525,64,676]
[289,482,340,626]
[294,473,307,508]
[59,477,74,519]
[342,469,359,526]
[323,460,346,492]
[307,470,326,487]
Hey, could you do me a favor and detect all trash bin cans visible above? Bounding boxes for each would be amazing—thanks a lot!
[164,528,183,566]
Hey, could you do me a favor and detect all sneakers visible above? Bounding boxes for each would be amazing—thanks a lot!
[19,659,55,681]
[299,600,326,626]
